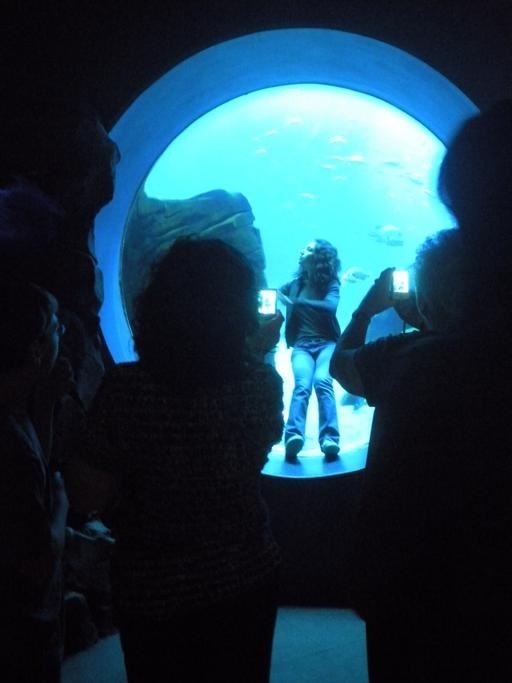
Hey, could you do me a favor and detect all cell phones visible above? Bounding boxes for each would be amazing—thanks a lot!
[389,269,410,300]
[254,289,277,320]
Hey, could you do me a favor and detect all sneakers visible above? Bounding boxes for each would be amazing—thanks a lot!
[284,435,304,462]
[319,438,340,458]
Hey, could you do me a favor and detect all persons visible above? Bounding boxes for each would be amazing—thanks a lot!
[1,94,512,682]
